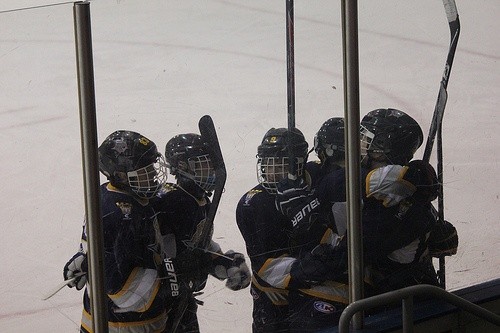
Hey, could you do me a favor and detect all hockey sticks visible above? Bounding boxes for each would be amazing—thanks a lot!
[163,114,228,333]
[420,0,461,165]
[436,80,449,294]
[39,267,95,303]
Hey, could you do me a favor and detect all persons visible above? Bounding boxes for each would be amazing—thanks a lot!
[63,130,251,333]
[236,108,459,333]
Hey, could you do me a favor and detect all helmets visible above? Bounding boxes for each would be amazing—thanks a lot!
[99,130,162,188]
[258,128,308,187]
[165,132,208,179]
[315,117,345,162]
[361,108,423,165]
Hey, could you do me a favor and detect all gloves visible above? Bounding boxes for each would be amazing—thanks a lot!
[224,251,251,291]
[63,252,87,290]
[274,180,323,238]
